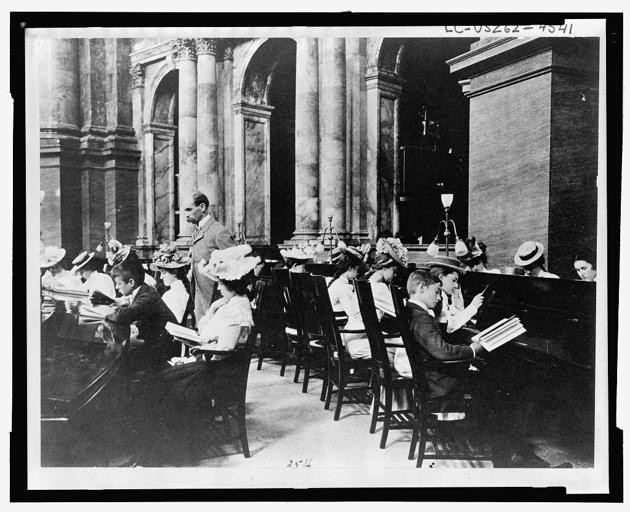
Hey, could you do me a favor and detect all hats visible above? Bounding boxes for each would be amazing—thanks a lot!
[279,243,318,263]
[335,234,370,270]
[103,238,132,274]
[197,242,263,284]
[68,247,96,274]
[374,235,408,271]
[423,254,467,273]
[512,240,547,270]
[147,238,193,271]
[39,244,68,269]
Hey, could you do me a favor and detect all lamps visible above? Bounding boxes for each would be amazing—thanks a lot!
[315,207,346,264]
[96,221,117,253]
[426,193,467,259]
[230,216,248,246]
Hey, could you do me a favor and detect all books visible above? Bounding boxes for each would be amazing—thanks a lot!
[77,297,118,322]
[165,320,202,346]
[471,314,527,354]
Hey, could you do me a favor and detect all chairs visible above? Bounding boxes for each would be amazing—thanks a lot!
[271,267,301,385]
[351,278,410,450]
[310,272,366,422]
[388,276,496,468]
[174,326,256,466]
[290,271,331,402]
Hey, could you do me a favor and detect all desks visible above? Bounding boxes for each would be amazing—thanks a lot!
[303,262,596,460]
[40,288,127,465]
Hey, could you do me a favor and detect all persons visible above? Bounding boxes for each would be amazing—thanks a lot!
[275,246,314,275]
[571,251,596,282]
[336,235,422,417]
[142,243,191,326]
[69,249,117,303]
[101,247,178,370]
[37,245,72,290]
[183,193,237,328]
[319,244,372,347]
[101,241,159,307]
[403,271,549,468]
[513,240,559,278]
[121,244,265,448]
[392,253,485,380]
[453,238,500,275]
